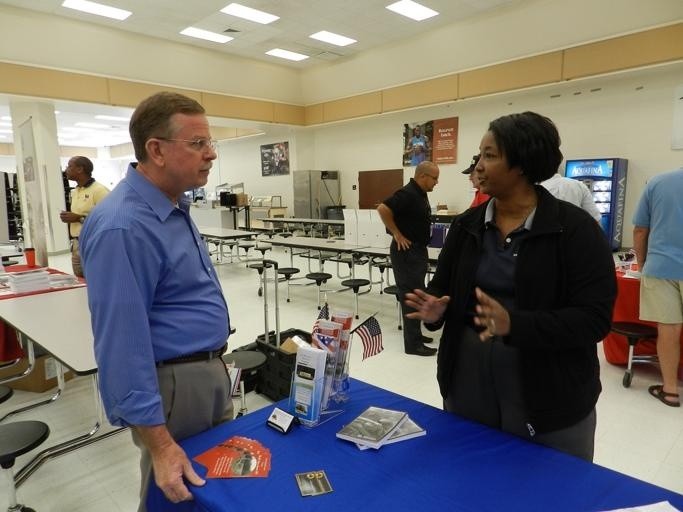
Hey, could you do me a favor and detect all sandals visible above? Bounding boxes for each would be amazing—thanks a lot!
[648,385,680,407]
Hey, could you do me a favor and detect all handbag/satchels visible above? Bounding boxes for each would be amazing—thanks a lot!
[232,328,294,396]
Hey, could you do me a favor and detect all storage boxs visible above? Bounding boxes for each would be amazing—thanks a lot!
[253,325,320,402]
[0,354,76,395]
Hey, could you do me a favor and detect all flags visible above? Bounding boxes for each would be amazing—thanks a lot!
[347,311,384,362]
[308,292,330,337]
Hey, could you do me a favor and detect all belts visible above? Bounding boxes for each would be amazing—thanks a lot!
[154,342,228,368]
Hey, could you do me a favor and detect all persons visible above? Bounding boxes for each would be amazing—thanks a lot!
[404,124,430,163]
[400,110,617,467]
[632,165,681,409]
[270,144,286,175]
[262,161,270,175]
[460,154,490,209]
[538,173,602,225]
[75,90,236,510]
[58,155,109,280]
[375,159,439,359]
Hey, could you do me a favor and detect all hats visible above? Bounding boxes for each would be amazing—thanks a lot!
[461,156,479,174]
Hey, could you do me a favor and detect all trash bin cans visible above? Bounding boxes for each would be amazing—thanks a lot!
[327,205,346,235]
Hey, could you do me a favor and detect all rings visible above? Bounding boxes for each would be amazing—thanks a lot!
[489,317,495,329]
[485,329,493,338]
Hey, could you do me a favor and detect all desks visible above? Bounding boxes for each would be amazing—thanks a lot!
[144,371,682,512]
[1,264,133,493]
[600,262,682,385]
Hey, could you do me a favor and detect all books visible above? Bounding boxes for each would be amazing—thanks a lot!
[333,403,409,450]
[341,416,428,450]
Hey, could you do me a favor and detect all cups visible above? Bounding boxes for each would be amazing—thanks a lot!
[23,248,35,267]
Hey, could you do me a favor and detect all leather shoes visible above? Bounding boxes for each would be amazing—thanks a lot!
[407,344,437,356]
[422,336,433,343]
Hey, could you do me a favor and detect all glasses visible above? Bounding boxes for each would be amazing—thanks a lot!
[143,136,214,153]
[424,173,438,181]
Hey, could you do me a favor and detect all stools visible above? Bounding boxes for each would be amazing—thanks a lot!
[0,383,15,409]
[607,321,656,388]
[187,199,408,332]
[0,418,52,512]
[218,348,270,418]
